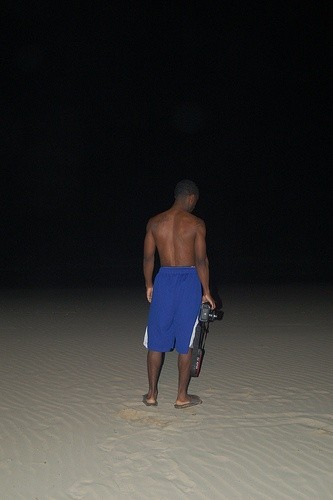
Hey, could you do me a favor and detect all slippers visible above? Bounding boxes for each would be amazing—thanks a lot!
[174,394,203,409]
[142,394,158,407]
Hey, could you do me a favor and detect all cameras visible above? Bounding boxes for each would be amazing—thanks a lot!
[199,303,218,322]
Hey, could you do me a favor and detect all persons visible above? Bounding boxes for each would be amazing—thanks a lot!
[143,180,215,408]
